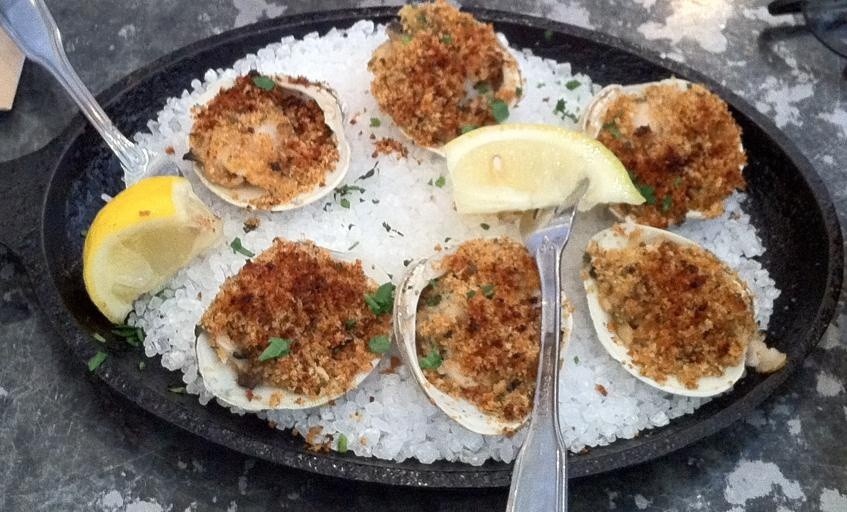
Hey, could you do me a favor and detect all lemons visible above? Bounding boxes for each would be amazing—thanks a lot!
[80,176,221,326]
[441,121,646,216]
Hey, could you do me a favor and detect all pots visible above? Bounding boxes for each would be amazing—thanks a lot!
[1,5,844,488]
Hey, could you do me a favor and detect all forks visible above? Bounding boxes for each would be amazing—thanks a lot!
[505,176,589,512]
